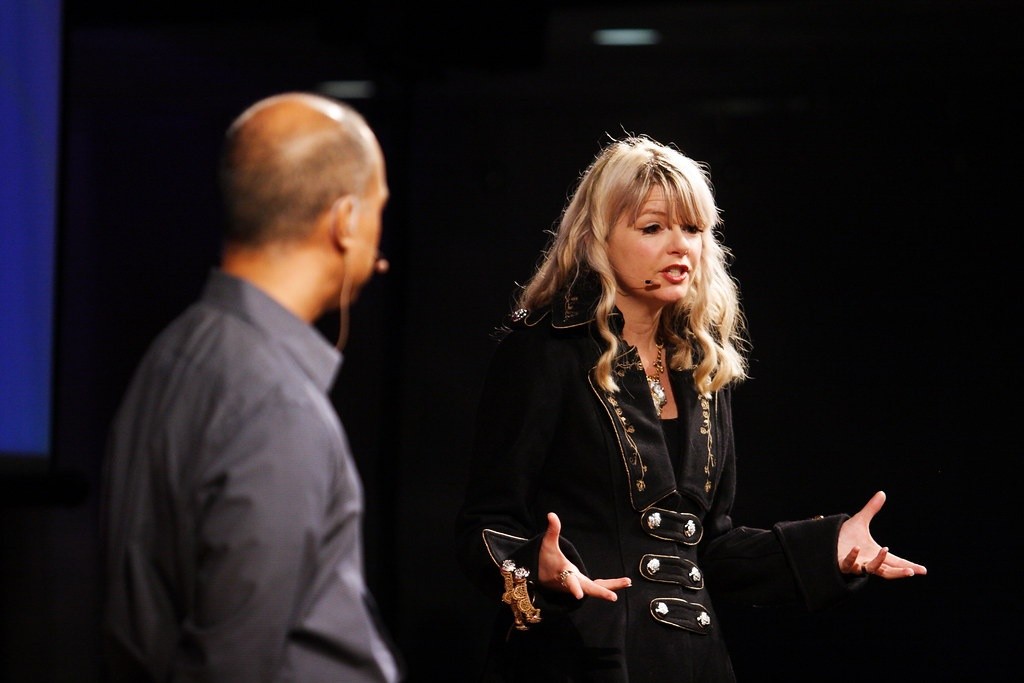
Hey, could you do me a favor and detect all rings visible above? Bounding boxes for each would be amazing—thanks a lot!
[861,562,867,574]
[557,569,570,591]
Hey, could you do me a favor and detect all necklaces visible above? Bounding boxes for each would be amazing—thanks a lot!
[646,342,667,418]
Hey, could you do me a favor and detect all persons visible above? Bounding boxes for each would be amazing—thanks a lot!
[104,92,398,683]
[482,137,927,683]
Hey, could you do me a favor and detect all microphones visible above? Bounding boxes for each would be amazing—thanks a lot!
[609,263,653,284]
[374,259,389,273]
